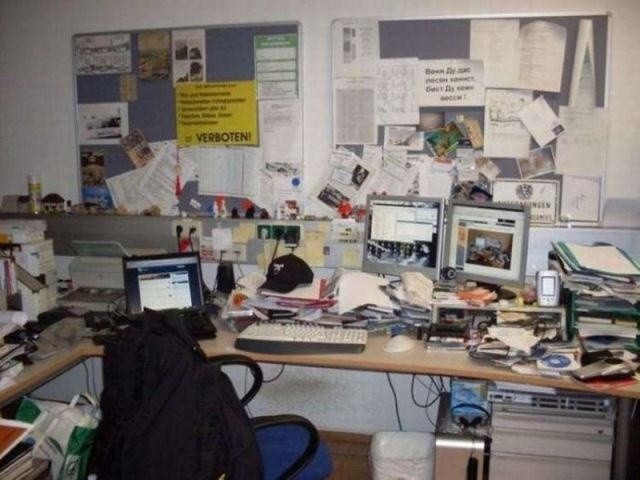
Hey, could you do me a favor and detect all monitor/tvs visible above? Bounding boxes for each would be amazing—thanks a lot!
[442,198,531,300]
[362,193,441,277]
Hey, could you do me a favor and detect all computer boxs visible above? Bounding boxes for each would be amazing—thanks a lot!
[433,392,489,480]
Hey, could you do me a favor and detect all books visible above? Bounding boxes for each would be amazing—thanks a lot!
[0,417,51,479]
[552,240,640,357]
[218,269,434,336]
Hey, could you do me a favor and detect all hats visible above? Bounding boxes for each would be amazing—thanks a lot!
[256,252,314,295]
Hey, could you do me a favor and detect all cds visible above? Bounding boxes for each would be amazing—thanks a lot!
[540,353,570,368]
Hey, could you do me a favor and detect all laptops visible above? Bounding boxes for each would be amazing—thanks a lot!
[122,251,216,338]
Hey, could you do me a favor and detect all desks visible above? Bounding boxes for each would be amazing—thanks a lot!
[1,300,638,480]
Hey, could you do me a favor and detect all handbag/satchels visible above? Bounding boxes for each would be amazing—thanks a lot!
[13,390,101,479]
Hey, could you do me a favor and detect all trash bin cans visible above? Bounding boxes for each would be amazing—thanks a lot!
[371,430,434,480]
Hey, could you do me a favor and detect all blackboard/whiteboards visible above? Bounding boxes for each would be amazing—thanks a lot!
[330,12,613,229]
[70,20,305,221]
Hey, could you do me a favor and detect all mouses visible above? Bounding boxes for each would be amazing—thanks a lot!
[383,332,415,353]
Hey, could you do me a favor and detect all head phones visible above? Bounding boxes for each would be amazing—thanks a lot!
[451,403,488,429]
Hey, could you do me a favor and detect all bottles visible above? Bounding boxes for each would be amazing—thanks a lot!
[212,198,226,218]
[28,173,43,215]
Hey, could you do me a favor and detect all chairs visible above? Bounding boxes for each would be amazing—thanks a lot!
[106,315,333,476]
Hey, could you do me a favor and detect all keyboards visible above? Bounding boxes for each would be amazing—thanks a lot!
[234,321,367,354]
